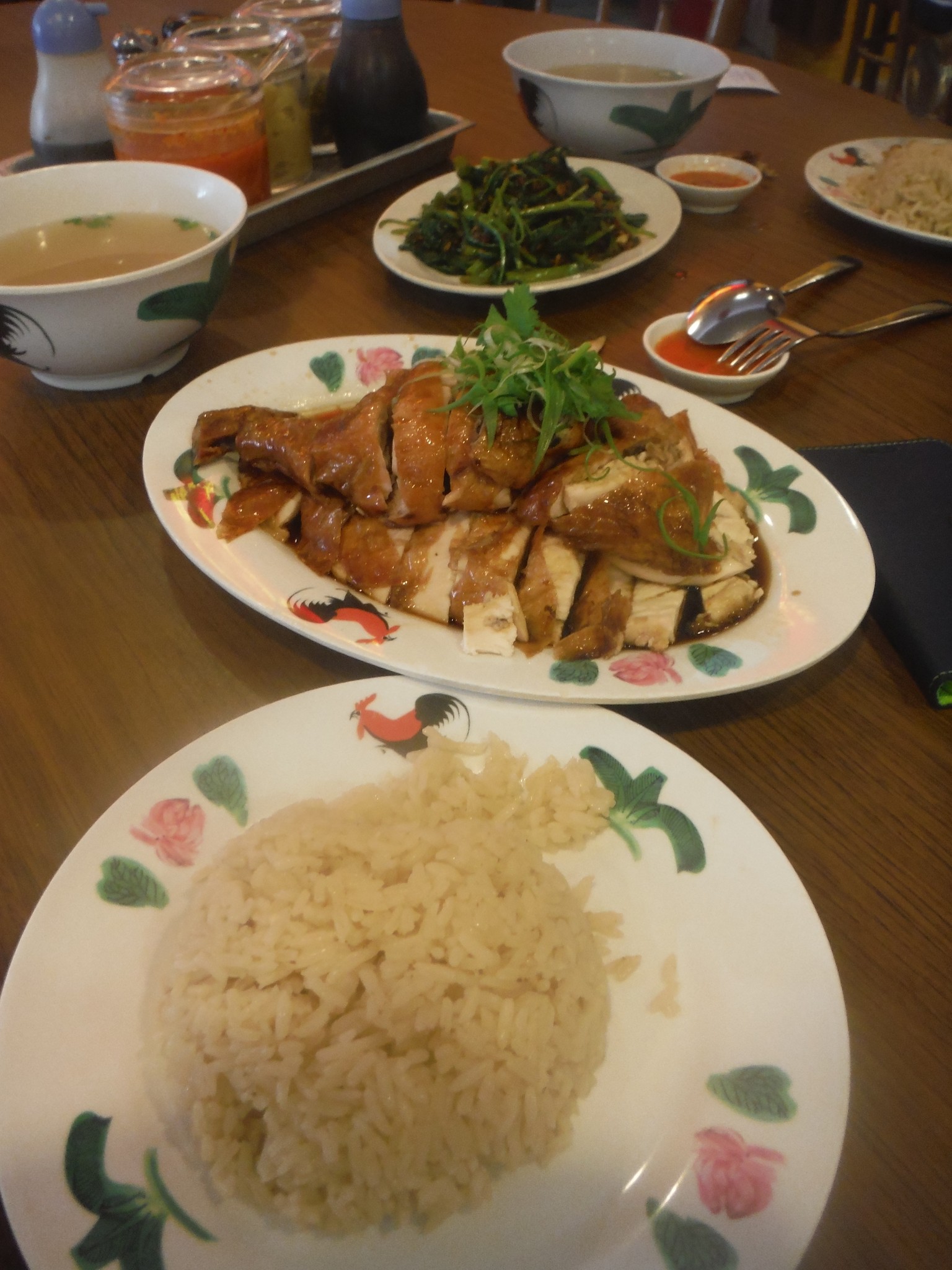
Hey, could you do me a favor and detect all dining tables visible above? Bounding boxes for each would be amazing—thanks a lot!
[2,2,952,1269]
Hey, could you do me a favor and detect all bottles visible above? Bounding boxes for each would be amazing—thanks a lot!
[23,0,129,162]
[326,0,429,173]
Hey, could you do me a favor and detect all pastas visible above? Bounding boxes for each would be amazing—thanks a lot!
[855,141,952,237]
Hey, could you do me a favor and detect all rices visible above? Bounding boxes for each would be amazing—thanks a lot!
[152,727,678,1237]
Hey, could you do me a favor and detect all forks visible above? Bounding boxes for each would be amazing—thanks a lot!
[718,298,952,377]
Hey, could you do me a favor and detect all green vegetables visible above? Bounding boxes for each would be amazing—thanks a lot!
[394,281,728,560]
[378,141,655,288]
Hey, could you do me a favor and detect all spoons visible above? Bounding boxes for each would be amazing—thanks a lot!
[688,252,862,344]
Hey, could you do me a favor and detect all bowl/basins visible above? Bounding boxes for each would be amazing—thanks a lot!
[642,312,790,405]
[0,153,248,393]
[653,153,764,215]
[500,26,732,169]
[106,1,346,212]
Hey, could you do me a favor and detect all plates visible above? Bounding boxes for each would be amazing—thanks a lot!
[144,334,875,707]
[0,662,854,1270]
[372,153,684,297]
[805,136,952,251]
